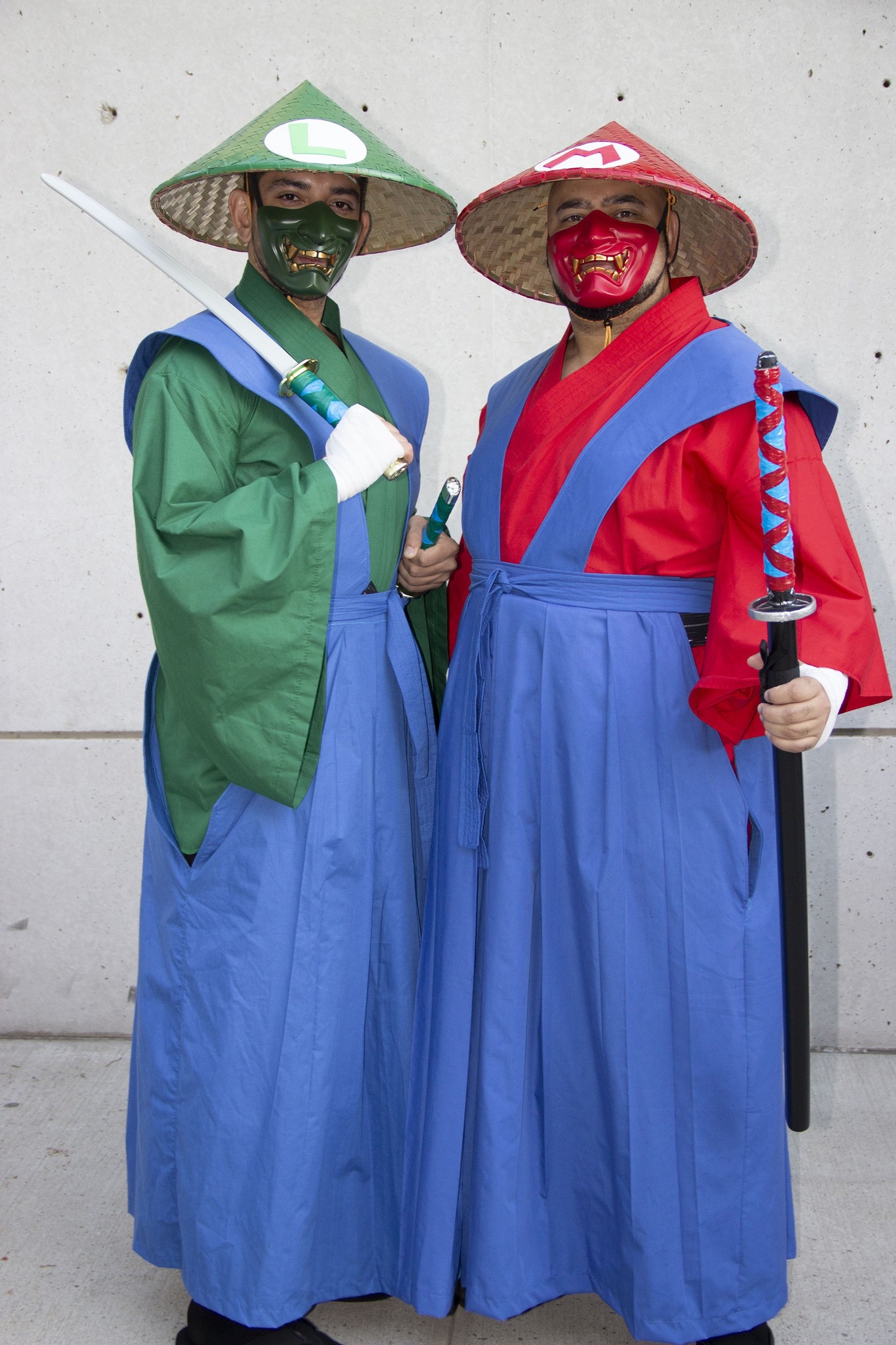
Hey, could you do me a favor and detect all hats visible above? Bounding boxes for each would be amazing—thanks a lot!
[148,78,460,257]
[454,120,760,304]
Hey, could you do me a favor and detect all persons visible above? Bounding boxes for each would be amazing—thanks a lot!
[120,89,893,1345]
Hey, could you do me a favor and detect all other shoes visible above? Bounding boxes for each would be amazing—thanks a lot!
[176,1296,338,1344]
